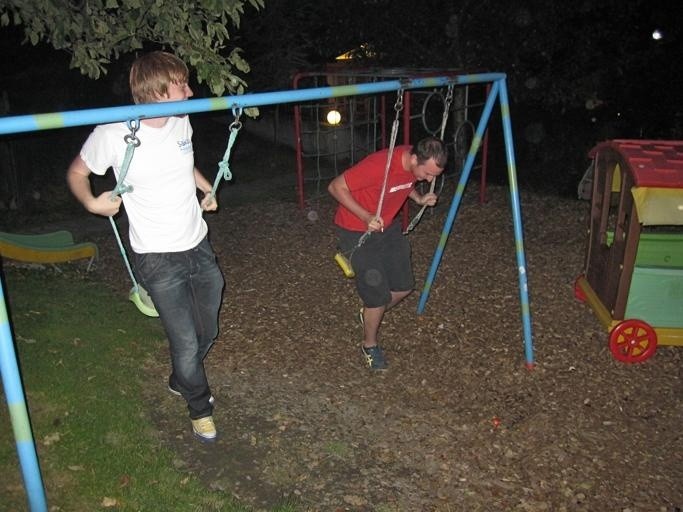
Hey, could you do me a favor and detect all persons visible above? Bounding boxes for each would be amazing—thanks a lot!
[66,50,226,446]
[327,136,448,371]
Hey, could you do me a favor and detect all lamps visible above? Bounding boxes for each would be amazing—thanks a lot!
[326,109,342,125]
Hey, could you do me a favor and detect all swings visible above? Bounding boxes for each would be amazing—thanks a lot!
[335,88,454,278]
[110,119,242,317]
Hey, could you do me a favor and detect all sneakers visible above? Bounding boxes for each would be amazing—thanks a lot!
[167,384,214,403]
[358,307,367,332]
[360,342,388,372]
[188,412,217,441]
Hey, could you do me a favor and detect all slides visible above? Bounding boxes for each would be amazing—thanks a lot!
[0,231,101,273]
